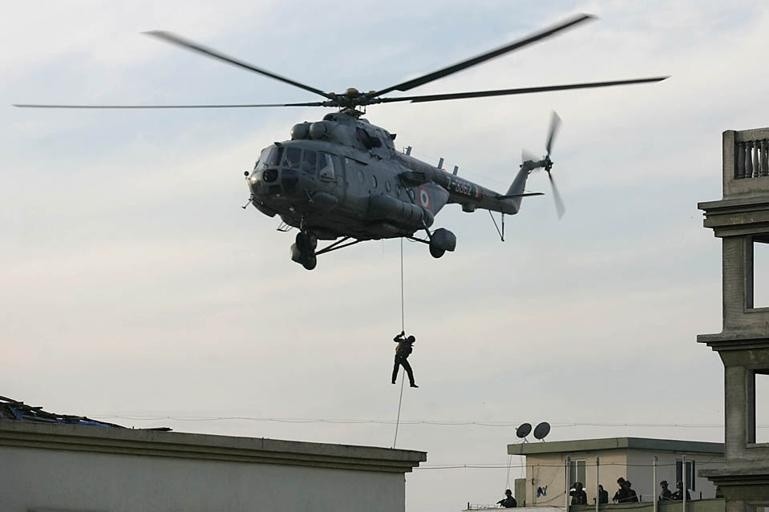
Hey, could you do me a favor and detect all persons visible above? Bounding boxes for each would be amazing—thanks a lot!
[657,480,671,502]
[499,489,517,508]
[672,480,691,500]
[391,330,419,388]
[598,484,608,504]
[569,482,587,505]
[615,477,638,502]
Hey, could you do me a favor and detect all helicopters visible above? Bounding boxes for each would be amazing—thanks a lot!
[10,14,671,270]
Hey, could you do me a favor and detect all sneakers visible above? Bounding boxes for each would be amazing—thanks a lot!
[410,383,420,388]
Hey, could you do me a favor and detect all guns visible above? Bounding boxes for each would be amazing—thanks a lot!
[496,499,505,507]
